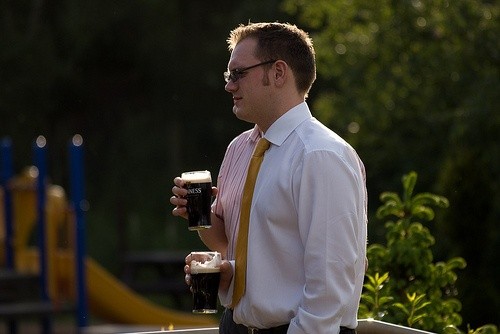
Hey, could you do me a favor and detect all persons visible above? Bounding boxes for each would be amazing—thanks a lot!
[185,20,368,334]
[170,123,262,334]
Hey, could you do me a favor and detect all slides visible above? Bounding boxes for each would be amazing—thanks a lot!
[82,256,216,329]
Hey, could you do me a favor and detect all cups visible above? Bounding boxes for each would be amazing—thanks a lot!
[182,171,212,231]
[189,252,222,314]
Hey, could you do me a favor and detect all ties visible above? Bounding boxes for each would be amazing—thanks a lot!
[230,137,270,307]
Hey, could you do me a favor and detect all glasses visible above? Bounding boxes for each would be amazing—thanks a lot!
[224,59,289,82]
